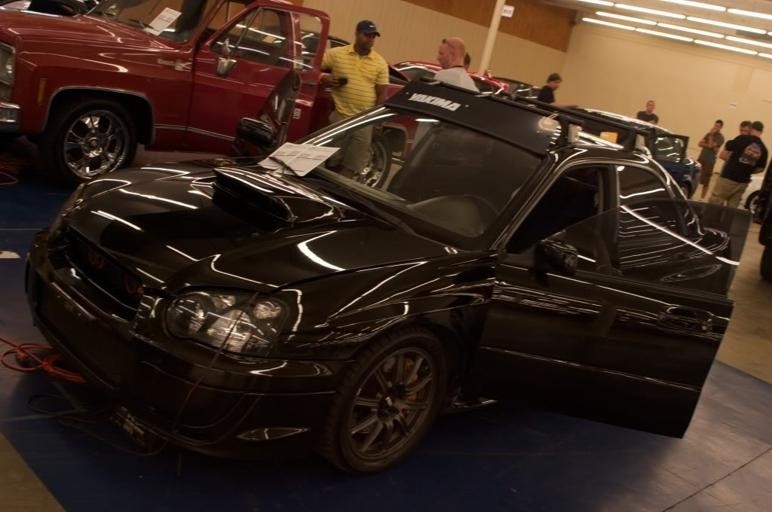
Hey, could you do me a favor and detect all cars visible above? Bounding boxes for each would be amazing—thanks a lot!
[562,107,703,200]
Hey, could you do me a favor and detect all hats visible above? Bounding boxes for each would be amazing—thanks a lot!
[357,20,380,37]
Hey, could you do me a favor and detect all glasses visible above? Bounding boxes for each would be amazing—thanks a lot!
[442,38,455,48]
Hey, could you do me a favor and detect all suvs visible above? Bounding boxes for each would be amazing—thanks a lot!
[21,72,757,477]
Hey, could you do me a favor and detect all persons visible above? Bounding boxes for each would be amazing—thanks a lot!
[537,73,561,112]
[695,120,725,202]
[408,37,480,145]
[463,53,471,70]
[708,120,768,204]
[637,100,659,124]
[717,121,751,162]
[316,18,390,179]
